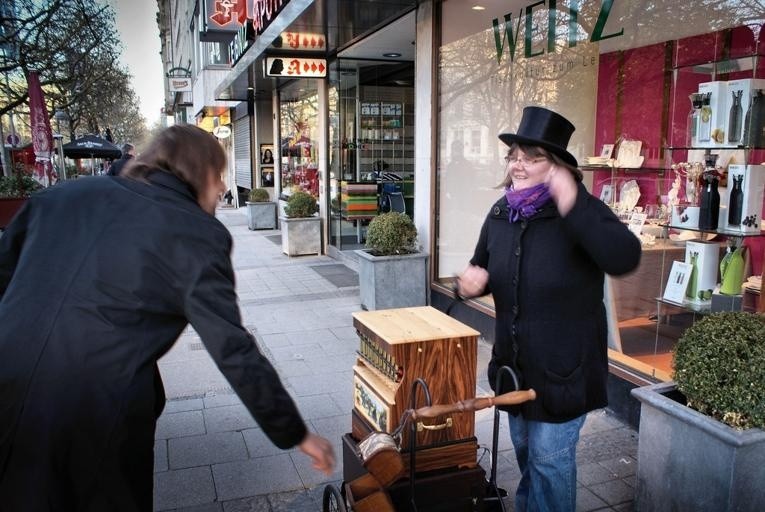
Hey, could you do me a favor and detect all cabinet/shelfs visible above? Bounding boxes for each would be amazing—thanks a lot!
[651,53,764,327]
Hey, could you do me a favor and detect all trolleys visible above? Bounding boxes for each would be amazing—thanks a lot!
[323,366,519,512]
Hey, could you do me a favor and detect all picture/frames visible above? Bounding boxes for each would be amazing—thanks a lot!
[261,167,274,187]
[260,143,274,164]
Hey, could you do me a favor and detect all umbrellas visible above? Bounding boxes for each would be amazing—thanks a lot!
[56,135,123,175]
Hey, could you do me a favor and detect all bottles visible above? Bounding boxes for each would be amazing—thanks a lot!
[685,91,711,148]
[697,173,719,233]
[726,173,744,226]
[720,246,744,296]
[725,90,741,143]
[686,250,698,300]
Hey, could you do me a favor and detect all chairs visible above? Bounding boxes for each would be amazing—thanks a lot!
[352,182,395,226]
[385,192,405,218]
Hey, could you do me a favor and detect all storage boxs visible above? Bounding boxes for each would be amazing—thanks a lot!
[680,77,764,315]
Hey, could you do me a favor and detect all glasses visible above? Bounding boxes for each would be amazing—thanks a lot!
[503,153,553,166]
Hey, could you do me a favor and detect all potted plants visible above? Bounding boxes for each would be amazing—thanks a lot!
[351,212,430,312]
[0,174,48,234]
[630,308,765,511]
[278,192,323,259]
[244,189,277,231]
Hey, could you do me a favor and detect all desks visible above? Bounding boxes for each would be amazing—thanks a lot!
[606,237,688,326]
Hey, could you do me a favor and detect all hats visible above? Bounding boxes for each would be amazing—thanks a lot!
[497,106,580,168]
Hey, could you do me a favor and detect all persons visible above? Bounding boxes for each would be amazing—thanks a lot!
[453,106,645,512]
[107,141,134,177]
[0,124,338,511]
[261,147,273,164]
